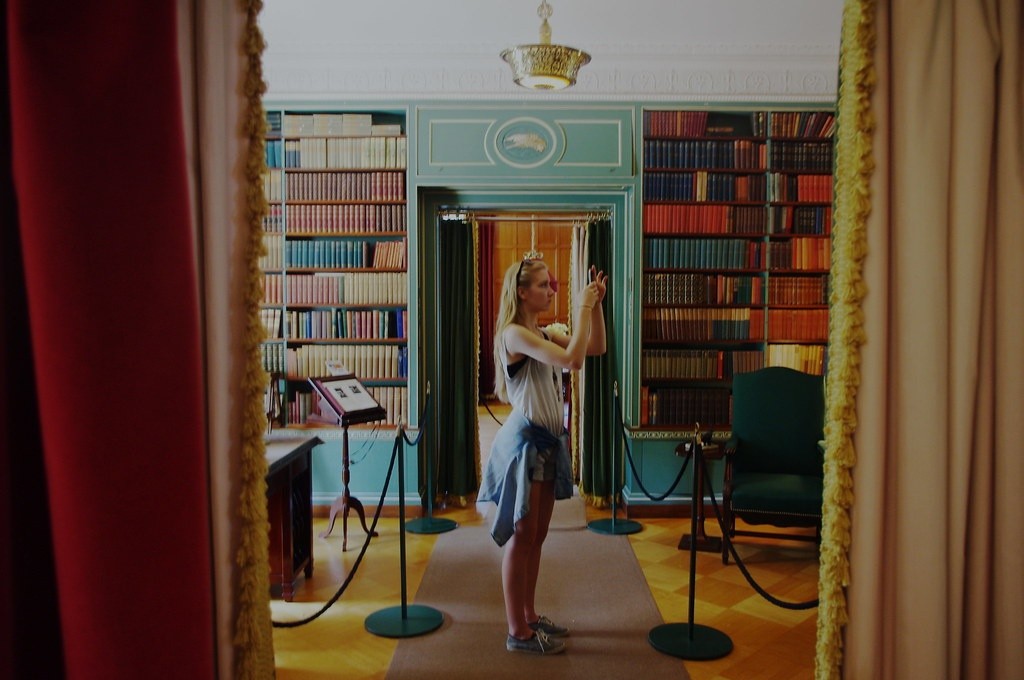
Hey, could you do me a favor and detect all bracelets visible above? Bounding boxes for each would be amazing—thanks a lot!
[580,303,593,312]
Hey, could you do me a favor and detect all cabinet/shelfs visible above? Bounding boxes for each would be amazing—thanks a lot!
[639,103,837,431]
[260,103,410,434]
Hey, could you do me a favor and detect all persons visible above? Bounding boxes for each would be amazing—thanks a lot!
[472,253,608,653]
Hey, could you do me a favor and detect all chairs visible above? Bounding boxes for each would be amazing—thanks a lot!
[718,365,826,566]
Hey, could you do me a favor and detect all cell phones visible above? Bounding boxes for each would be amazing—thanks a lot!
[588,269,591,284]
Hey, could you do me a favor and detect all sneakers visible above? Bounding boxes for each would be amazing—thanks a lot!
[506,629,566,655]
[527,615,570,638]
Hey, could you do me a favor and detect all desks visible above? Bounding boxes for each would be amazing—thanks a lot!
[265,434,323,604]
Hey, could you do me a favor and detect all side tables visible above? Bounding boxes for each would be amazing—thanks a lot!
[675,438,727,554]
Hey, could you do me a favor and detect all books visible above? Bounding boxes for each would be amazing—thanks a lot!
[637,110,835,439]
[260,107,413,431]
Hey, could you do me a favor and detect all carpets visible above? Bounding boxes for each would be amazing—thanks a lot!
[384,491,692,680]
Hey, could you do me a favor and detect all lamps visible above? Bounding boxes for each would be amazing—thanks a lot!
[500,0,592,90]
